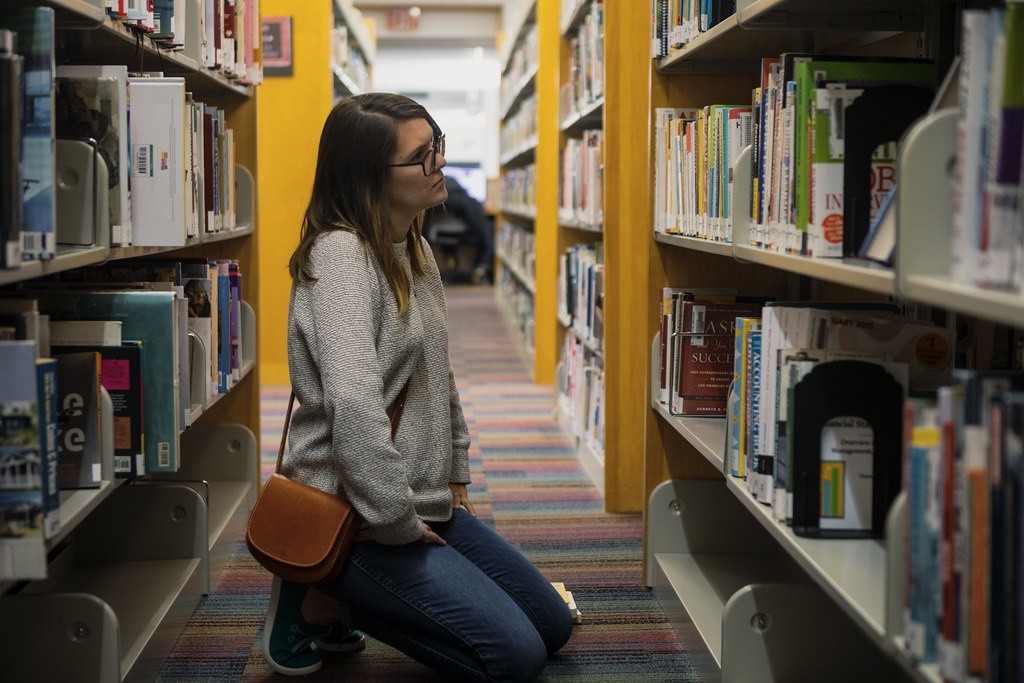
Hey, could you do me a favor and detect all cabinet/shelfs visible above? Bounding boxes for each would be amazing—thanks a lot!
[495,0,1023,683]
[0,0,378,683]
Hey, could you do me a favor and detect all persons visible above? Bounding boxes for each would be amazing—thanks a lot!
[261,93,573,683]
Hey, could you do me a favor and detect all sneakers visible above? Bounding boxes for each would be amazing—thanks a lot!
[315,601,366,652]
[262,573,321,676]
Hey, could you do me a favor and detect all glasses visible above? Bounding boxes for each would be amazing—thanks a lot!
[386,133,446,177]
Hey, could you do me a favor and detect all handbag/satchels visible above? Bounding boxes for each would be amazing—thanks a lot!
[245,473,361,587]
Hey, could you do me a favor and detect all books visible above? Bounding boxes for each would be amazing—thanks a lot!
[550,581,582,624]
[650,0,1024,683]
[498,0,604,462]
[0,0,259,567]
